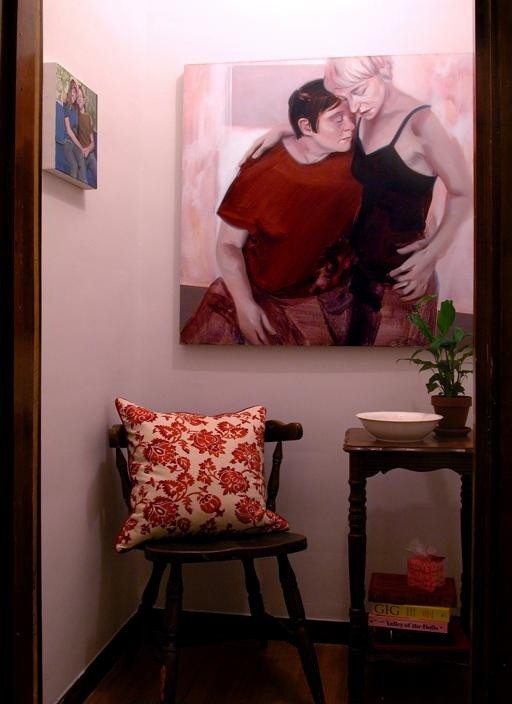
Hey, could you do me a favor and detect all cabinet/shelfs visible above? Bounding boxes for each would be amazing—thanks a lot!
[343,422,477,704]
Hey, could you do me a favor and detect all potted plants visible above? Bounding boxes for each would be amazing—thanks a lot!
[395,293,473,436]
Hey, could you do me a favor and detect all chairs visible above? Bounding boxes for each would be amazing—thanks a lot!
[108,420,327,704]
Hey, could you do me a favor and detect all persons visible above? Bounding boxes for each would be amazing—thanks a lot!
[62,81,96,182]
[320,56,474,344]
[181,81,362,343]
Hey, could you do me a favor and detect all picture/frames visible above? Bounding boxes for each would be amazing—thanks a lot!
[174,56,480,348]
[41,61,105,193]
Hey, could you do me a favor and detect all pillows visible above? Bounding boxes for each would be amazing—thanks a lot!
[107,391,289,555]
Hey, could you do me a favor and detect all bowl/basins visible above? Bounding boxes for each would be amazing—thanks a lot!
[356,410,443,442]
